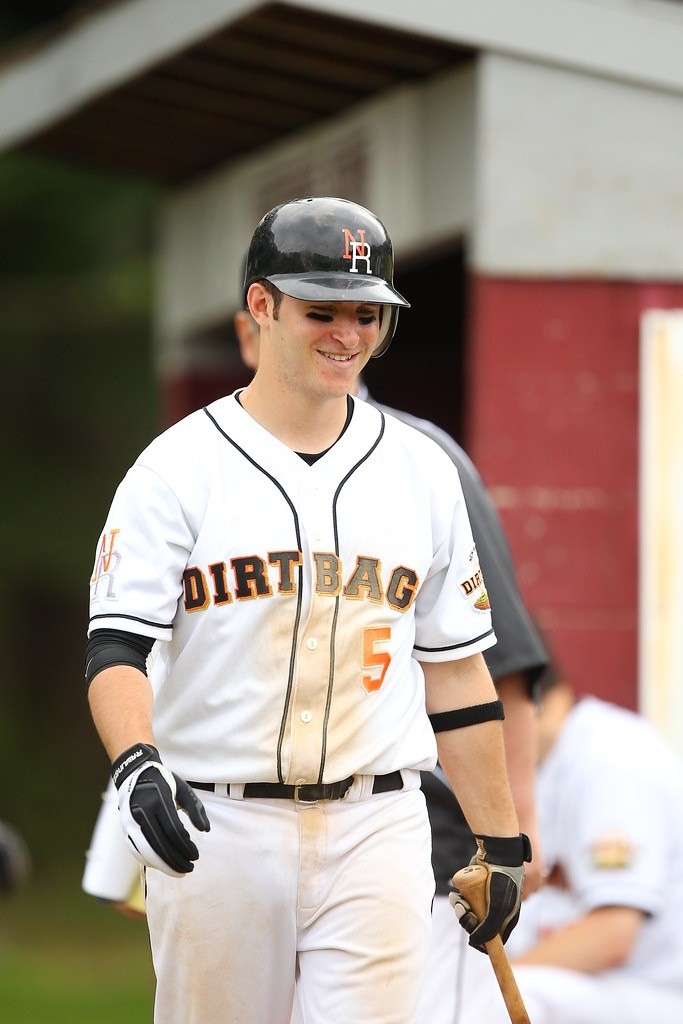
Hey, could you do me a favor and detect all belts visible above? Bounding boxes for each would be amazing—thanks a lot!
[186,770,403,804]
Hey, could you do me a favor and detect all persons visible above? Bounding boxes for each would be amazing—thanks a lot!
[84,197,534,1024]
[79,244,681,1024]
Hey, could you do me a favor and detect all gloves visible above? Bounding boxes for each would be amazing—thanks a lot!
[111,743,210,878]
[449,833,532,954]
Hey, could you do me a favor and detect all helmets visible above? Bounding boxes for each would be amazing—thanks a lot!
[243,196,411,358]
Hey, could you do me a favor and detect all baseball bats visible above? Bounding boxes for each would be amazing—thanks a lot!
[451,864,534,1024]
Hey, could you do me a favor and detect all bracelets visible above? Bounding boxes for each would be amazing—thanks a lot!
[429,700,506,734]
